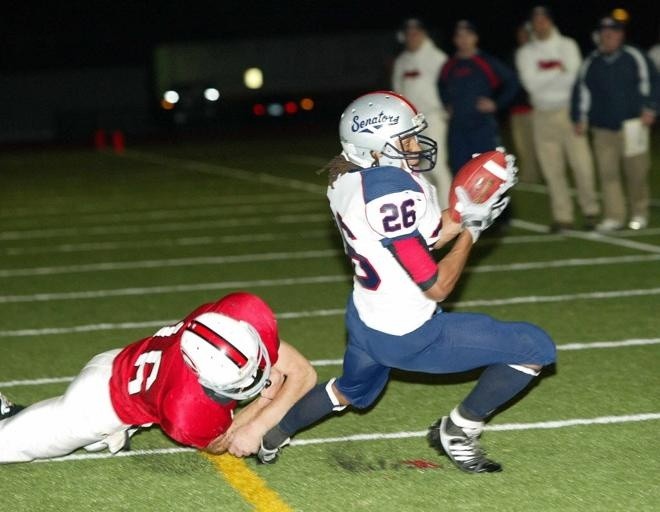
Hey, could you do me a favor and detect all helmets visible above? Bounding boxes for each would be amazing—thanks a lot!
[339,90,437,174]
[181,313,271,401]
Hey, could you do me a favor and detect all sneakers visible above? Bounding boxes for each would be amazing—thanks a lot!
[257,437,290,464]
[426,416,502,473]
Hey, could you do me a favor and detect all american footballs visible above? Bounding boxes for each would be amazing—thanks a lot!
[449,151,507,223]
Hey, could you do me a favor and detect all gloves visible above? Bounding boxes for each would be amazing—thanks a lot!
[454,146,519,244]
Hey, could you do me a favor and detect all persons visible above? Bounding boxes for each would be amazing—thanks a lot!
[247,89,558,475]
[389,4,660,234]
[1,290,320,465]
